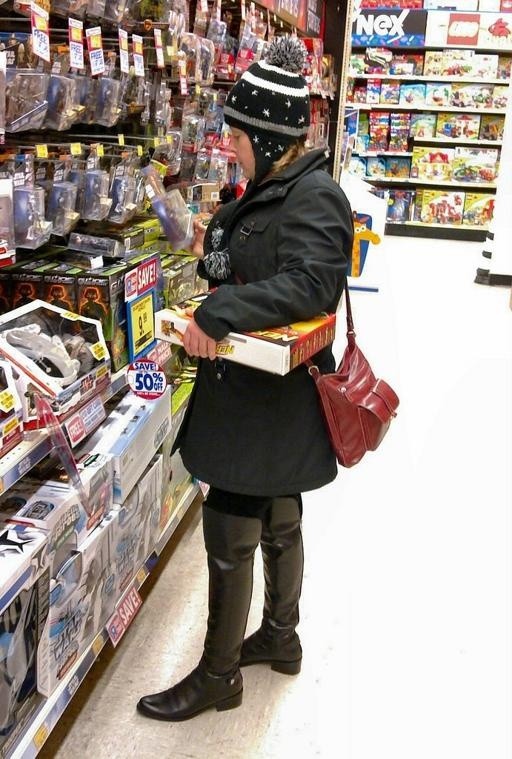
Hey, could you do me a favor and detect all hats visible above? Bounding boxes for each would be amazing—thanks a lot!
[223,35,312,180]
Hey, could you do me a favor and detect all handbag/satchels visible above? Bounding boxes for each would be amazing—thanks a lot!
[313,339,399,469]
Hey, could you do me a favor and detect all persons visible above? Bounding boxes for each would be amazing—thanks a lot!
[135,35,356,725]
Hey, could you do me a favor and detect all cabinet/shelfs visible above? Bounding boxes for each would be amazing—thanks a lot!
[332,0,512,242]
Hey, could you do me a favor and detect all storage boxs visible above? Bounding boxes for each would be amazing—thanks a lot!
[153,285,336,377]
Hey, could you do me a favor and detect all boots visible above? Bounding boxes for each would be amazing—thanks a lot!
[135,500,262,722]
[239,498,322,674]
